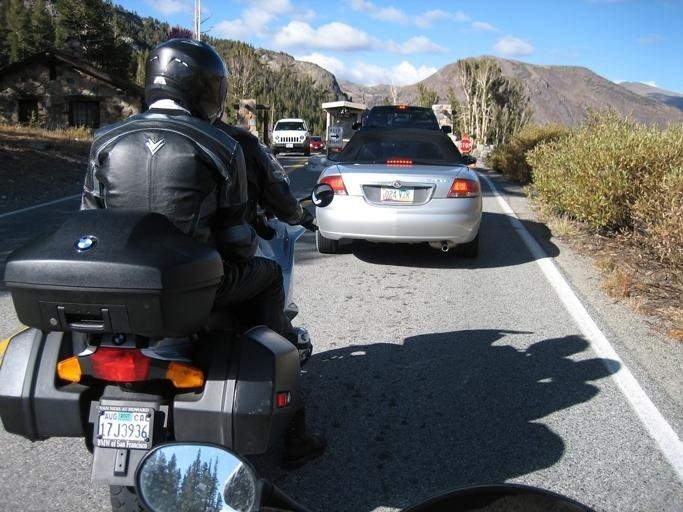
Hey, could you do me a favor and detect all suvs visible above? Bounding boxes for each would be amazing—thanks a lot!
[268,117,310,157]
[351,105,451,135]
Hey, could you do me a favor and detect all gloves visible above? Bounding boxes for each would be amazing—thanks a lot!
[298,206,313,226]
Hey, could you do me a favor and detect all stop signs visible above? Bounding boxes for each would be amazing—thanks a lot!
[458,137,473,153]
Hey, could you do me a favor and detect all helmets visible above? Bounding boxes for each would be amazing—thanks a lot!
[144,38,228,125]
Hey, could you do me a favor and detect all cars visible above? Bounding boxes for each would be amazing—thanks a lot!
[308,135,324,152]
[308,122,487,260]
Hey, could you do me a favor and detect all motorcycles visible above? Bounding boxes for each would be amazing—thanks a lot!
[0,181,336,512]
[132,437,597,512]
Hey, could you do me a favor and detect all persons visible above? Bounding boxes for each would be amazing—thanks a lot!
[211,117,314,351]
[77,38,286,332]
